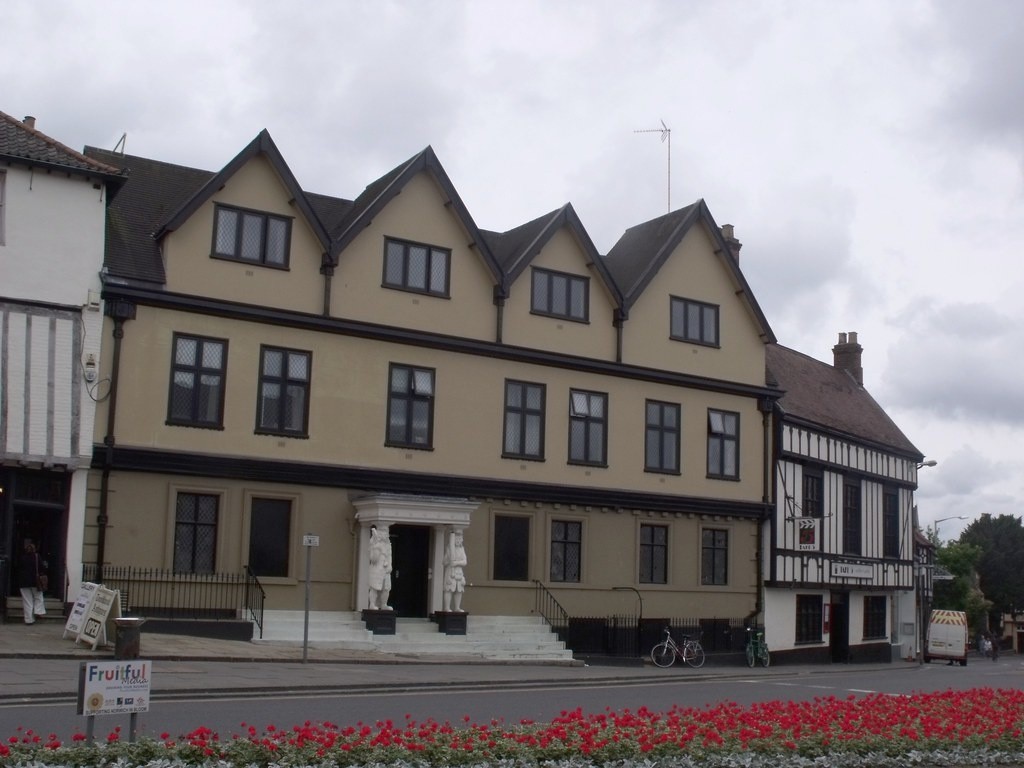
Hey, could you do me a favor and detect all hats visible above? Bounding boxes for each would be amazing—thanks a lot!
[24,544,35,552]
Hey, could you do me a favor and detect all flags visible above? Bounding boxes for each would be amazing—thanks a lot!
[797,516,821,553]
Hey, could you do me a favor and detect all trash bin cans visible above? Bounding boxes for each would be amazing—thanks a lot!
[110,617,147,660]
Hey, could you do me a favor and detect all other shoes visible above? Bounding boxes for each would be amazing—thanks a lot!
[36,614,43,620]
[28,622,35,624]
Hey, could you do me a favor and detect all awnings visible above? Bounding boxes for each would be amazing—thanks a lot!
[913,529,942,553]
[933,566,955,581]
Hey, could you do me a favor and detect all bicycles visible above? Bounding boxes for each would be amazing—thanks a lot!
[744,627,771,668]
[650,626,705,668]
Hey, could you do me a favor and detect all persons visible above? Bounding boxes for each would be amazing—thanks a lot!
[368,527,394,610]
[978,634,999,661]
[442,533,466,612]
[17,543,47,626]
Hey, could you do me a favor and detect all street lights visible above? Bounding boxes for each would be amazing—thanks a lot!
[934,516,966,554]
[917,461,937,469]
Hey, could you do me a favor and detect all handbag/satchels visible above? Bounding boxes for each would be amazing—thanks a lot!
[37,575,48,591]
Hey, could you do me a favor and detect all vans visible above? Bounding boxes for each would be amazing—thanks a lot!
[924,610,969,666]
